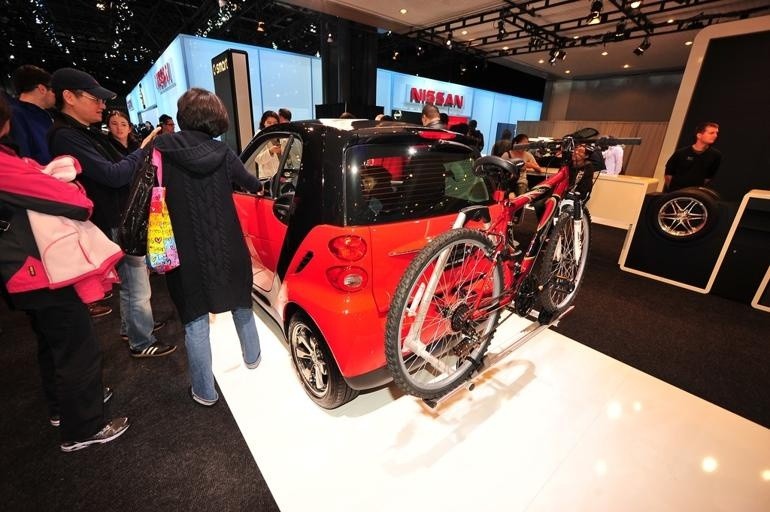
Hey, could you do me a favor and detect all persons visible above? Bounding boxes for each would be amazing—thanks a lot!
[0,64,175,452]
[662,123,719,194]
[600,141,624,175]
[277,108,292,125]
[150,87,265,408]
[491,129,513,158]
[500,133,540,210]
[252,110,300,179]
[343,105,484,211]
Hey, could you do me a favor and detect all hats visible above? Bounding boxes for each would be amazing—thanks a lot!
[50,68,118,101]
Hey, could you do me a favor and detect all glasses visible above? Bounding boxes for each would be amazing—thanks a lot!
[79,94,106,105]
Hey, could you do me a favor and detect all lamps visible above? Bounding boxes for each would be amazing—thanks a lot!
[384,0,650,64]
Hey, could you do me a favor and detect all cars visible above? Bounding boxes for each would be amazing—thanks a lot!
[526,136,562,159]
[230,114,517,414]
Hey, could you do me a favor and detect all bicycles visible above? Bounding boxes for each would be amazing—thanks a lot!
[381,129,644,403]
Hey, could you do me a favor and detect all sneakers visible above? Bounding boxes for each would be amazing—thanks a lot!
[120,319,165,341]
[87,302,112,318]
[60,415,132,452]
[130,341,178,357]
[49,384,113,426]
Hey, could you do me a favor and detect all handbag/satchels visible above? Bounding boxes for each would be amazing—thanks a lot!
[145,146,180,275]
[117,137,158,257]
